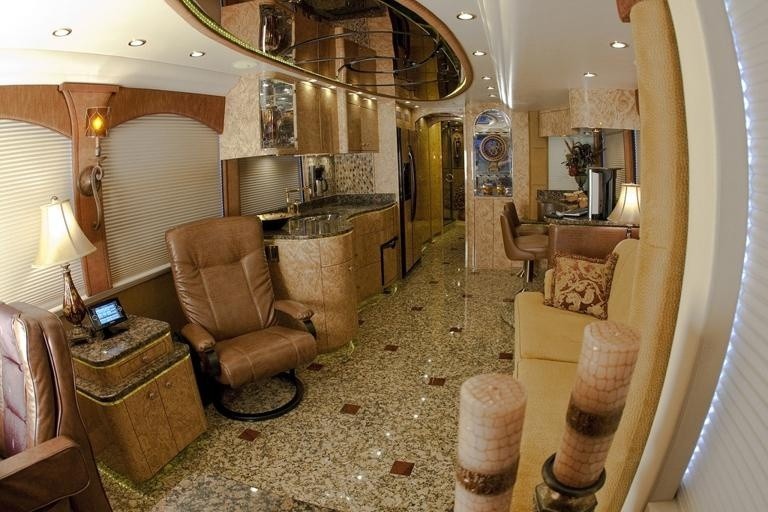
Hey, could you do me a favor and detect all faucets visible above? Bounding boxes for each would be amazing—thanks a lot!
[286,186,312,203]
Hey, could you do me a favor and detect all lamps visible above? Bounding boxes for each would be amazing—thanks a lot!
[607,182,640,239]
[76,105,113,231]
[30,195,99,347]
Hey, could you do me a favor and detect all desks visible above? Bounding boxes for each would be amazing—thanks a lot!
[536,197,640,270]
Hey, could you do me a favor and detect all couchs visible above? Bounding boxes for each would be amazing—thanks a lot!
[507,238,639,511]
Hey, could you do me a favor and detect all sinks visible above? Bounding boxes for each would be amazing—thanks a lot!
[299,213,340,223]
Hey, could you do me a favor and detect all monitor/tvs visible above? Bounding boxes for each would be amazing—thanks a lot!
[84,296,129,340]
[588,167,624,222]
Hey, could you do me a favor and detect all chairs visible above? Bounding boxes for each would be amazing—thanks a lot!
[164,216,318,423]
[500,202,549,295]
[0,300,115,512]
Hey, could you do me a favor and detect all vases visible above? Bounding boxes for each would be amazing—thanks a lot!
[574,175,587,191]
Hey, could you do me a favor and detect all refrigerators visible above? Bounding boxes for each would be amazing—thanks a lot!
[397,126,421,273]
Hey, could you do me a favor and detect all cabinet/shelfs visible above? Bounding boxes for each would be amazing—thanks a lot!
[221,0,377,93]
[63,312,210,485]
[264,206,400,353]
[219,72,379,161]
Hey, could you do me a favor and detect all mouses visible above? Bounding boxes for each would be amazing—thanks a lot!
[544,211,559,219]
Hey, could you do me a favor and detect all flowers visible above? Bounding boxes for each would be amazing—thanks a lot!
[561,137,607,177]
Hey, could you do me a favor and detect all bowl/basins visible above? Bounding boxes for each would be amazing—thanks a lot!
[256,213,292,232]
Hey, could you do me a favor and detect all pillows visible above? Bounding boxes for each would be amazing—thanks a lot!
[543,249,619,320]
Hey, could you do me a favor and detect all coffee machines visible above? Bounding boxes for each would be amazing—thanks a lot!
[309,165,328,197]
